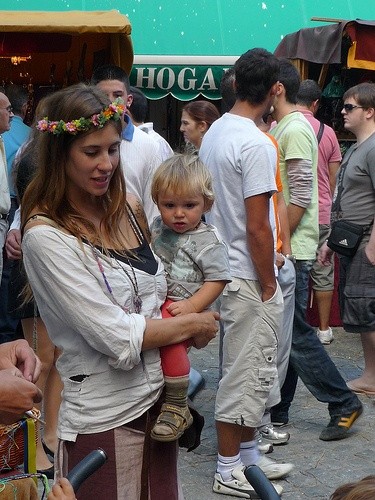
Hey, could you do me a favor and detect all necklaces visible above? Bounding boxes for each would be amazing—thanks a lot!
[66,194,143,314]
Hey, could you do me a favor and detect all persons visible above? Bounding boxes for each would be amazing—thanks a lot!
[191,47,295,500]
[329,82,375,398]
[178,100,219,158]
[148,153,232,443]
[17,84,221,500]
[221,58,364,453]
[145,153,234,441]
[0,339,51,425]
[47,478,78,500]
[0,63,175,482]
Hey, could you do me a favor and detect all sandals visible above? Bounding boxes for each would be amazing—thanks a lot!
[151,403,193,441]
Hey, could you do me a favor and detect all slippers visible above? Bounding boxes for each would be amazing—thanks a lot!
[346,382,375,394]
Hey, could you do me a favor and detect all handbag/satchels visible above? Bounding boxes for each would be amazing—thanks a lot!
[0,473,49,500]
[178,406,204,453]
[327,221,366,258]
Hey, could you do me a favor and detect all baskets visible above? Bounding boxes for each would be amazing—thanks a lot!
[0,407,41,473]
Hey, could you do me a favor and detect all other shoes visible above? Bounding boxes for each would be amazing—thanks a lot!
[189,378,205,399]
[42,441,54,457]
[36,466,54,480]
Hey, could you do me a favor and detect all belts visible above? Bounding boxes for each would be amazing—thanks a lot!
[2,213,9,220]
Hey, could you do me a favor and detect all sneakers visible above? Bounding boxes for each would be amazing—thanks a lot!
[213,462,283,500]
[270,410,288,425]
[253,428,274,454]
[258,423,290,444]
[319,393,363,441]
[255,456,294,479]
[317,326,333,344]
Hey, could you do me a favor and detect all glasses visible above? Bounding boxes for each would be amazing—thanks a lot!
[344,104,369,111]
[0,106,13,112]
